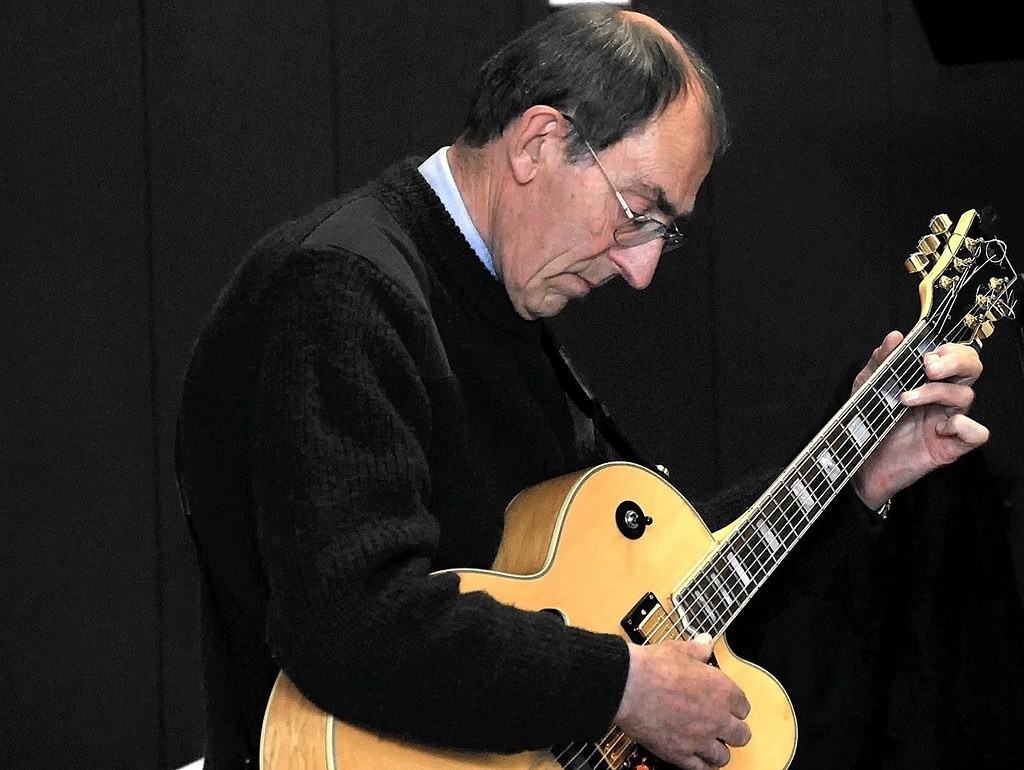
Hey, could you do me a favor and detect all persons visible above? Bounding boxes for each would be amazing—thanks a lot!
[176,4,990,770]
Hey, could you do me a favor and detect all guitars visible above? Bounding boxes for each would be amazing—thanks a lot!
[259,206,1018,770]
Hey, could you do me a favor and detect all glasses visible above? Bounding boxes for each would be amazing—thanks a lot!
[562,113,687,253]
